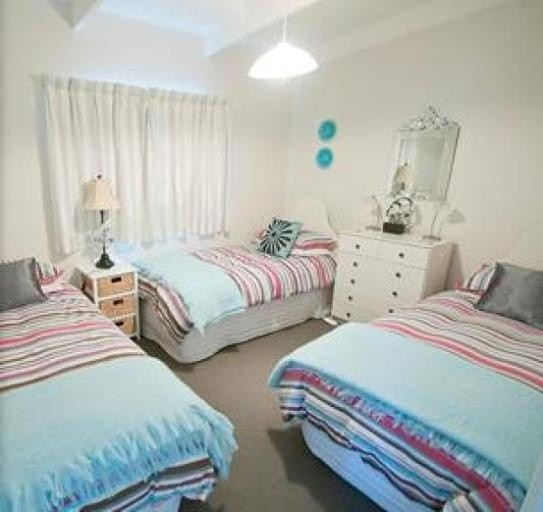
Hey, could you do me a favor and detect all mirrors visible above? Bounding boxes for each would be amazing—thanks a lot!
[387,101,461,201]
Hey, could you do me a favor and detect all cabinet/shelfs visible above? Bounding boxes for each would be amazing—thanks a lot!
[331,227,453,321]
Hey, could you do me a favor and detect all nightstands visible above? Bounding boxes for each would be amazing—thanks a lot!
[77,266,141,341]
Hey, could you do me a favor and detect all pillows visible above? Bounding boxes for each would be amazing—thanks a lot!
[460,257,543,331]
[256,213,337,258]
[0,256,71,313]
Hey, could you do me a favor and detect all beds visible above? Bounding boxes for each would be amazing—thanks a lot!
[0,282,217,512]
[126,198,337,365]
[288,221,543,512]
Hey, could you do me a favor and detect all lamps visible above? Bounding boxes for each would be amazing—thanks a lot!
[395,162,415,192]
[84,175,119,269]
[247,11,319,80]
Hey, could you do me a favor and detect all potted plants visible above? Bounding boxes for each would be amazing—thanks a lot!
[383,197,414,234]
